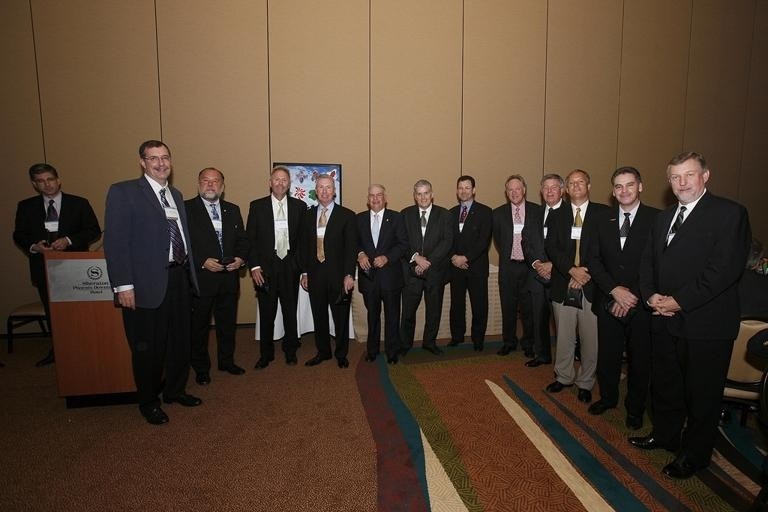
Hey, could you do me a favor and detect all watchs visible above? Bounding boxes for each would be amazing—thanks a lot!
[346,274,354,279]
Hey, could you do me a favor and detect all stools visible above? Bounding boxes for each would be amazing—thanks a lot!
[8,304,49,354]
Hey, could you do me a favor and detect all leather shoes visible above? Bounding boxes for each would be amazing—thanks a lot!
[163,394,201,407]
[255,353,274,368]
[623,408,710,479]
[422,336,484,356]
[286,354,298,365]
[35,353,54,367]
[219,365,246,375]
[546,379,618,415]
[335,352,349,369]
[141,406,169,425]
[305,353,332,366]
[196,373,211,385]
[365,348,410,364]
[499,340,551,367]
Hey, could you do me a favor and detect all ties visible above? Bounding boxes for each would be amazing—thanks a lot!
[544,206,686,267]
[316,207,328,263]
[210,203,223,258]
[159,188,186,264]
[372,214,380,248]
[276,202,288,260]
[459,206,468,222]
[420,211,427,227]
[46,200,60,247]
[514,207,523,262]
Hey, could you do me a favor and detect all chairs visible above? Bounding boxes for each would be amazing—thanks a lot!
[709,372,768,467]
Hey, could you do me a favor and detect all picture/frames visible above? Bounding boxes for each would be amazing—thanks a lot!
[273,162,341,206]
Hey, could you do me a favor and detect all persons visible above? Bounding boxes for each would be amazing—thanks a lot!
[491,175,540,359]
[181,168,246,386]
[627,149,751,481]
[298,173,356,369]
[521,173,582,367]
[446,175,493,352]
[103,139,203,425]
[586,166,666,430]
[398,178,453,359]
[546,169,610,404]
[357,183,409,366]
[11,163,102,367]
[242,167,307,370]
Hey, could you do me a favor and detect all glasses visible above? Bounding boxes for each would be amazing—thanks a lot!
[143,156,171,161]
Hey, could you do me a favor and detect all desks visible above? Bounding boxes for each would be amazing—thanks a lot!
[46,251,141,409]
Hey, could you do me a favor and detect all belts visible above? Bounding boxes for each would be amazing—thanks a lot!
[168,256,190,270]
[509,259,526,265]
